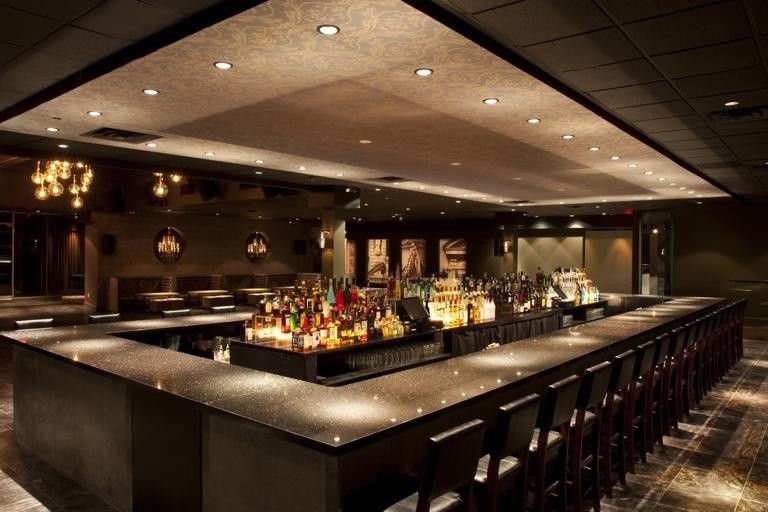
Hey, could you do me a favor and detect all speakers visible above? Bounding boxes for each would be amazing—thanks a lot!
[295,239,306,256]
[101,235,115,254]
[643,234,650,265]
[494,230,504,255]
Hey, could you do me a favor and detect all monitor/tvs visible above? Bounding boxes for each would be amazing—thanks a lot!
[399,296,431,322]
[552,285,568,299]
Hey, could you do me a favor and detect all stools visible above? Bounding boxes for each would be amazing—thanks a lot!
[382,298,746,511]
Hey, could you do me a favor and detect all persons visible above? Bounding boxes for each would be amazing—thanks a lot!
[401,242,426,283]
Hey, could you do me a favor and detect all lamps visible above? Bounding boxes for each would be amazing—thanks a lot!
[317,227,330,253]
[28,158,94,211]
[150,171,183,199]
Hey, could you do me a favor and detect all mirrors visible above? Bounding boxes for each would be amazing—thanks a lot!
[245,230,273,266]
[152,227,185,266]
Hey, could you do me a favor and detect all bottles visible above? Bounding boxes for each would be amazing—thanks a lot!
[536,265,599,306]
[411,271,496,325]
[252,276,411,350]
